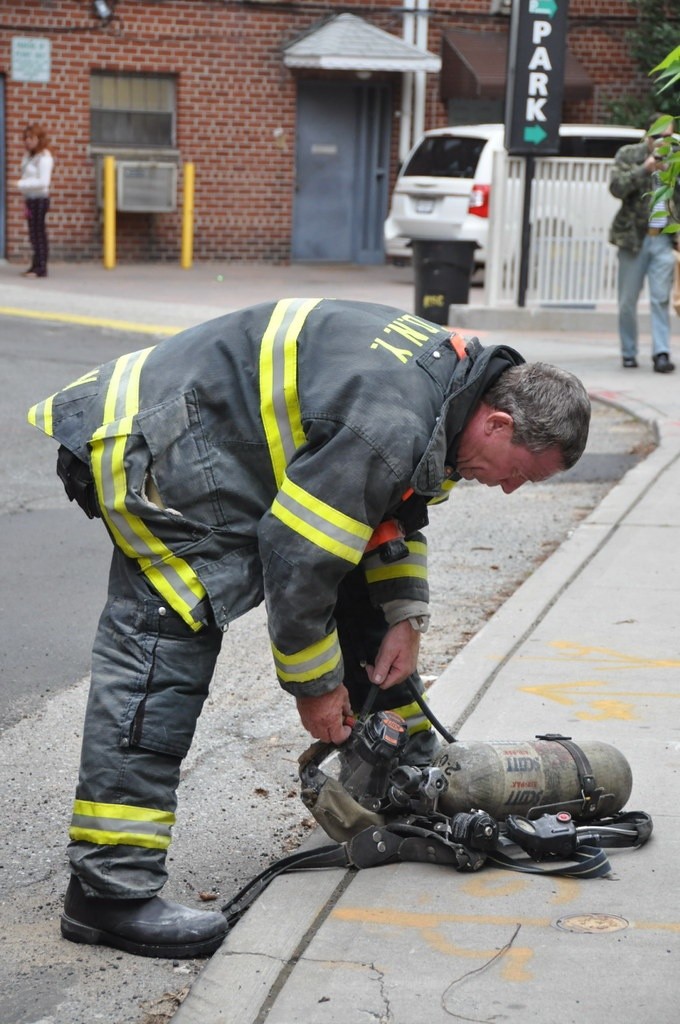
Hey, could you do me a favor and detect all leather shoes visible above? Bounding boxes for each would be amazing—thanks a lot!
[54,873,228,958]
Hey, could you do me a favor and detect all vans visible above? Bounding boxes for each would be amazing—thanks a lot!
[380,127,680,285]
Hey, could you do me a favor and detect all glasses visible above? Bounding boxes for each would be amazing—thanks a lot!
[652,134,671,141]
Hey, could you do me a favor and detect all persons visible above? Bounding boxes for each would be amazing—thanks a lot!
[60,296,590,955]
[13,122,55,277]
[606,112,680,374]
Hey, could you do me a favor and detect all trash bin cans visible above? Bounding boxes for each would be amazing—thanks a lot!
[406,238,483,325]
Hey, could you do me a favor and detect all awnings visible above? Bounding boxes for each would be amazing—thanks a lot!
[283,13,442,75]
[440,27,595,101]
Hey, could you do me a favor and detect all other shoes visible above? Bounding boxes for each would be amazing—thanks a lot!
[21,269,48,278]
[654,355,674,372]
[623,358,637,368]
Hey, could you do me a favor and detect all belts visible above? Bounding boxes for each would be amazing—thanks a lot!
[647,228,672,235]
